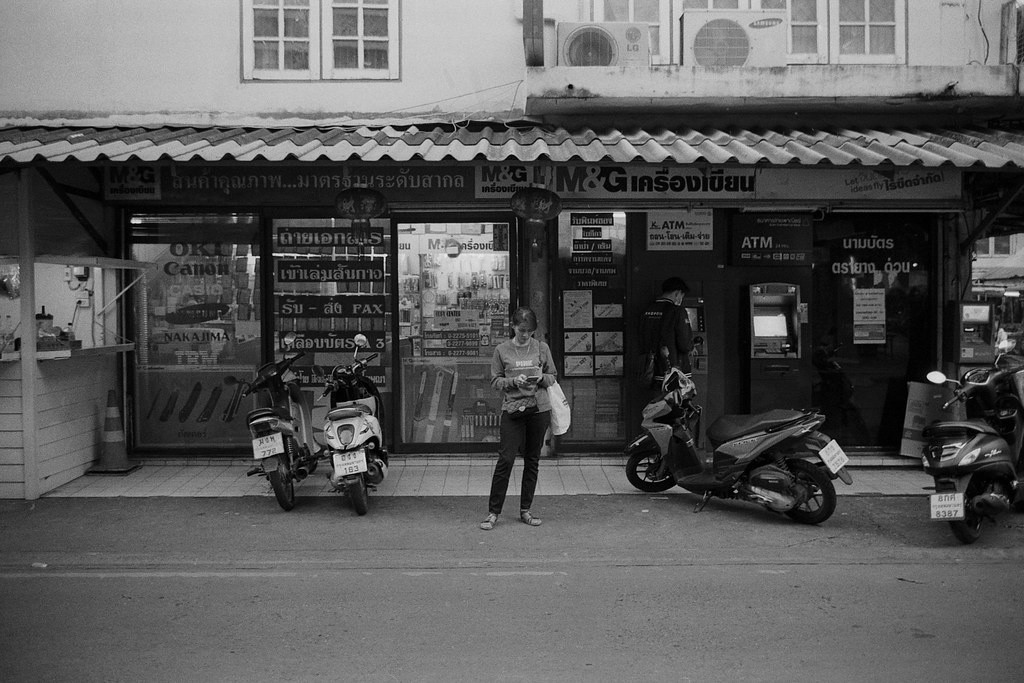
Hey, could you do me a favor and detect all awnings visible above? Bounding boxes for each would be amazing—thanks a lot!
[0,115,1024,168]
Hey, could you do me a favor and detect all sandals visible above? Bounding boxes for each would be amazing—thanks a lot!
[480,512,498,530]
[520,509,542,525]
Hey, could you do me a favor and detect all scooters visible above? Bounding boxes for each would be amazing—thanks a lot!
[621,346,854,527]
[919,338,1024,545]
[312,334,390,516]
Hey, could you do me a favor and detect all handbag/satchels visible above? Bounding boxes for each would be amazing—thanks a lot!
[506,396,539,419]
[639,346,674,381]
[547,380,571,436]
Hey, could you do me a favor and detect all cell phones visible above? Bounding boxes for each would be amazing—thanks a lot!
[524,376,539,383]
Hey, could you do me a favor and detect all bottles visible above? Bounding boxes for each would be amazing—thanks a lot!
[63,323,74,340]
[0,315,15,353]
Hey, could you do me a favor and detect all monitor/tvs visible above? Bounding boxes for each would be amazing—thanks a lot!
[754,315,787,336]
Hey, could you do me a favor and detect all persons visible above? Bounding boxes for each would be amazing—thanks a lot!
[481,306,556,530]
[812,327,838,387]
[639,277,694,423]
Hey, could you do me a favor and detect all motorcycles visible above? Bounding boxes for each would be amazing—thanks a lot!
[223,329,325,513]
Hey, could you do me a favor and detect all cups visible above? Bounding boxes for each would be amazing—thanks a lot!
[35,313,54,336]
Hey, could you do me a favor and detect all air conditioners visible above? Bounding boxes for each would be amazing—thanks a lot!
[680,9,788,65]
[558,23,652,66]
